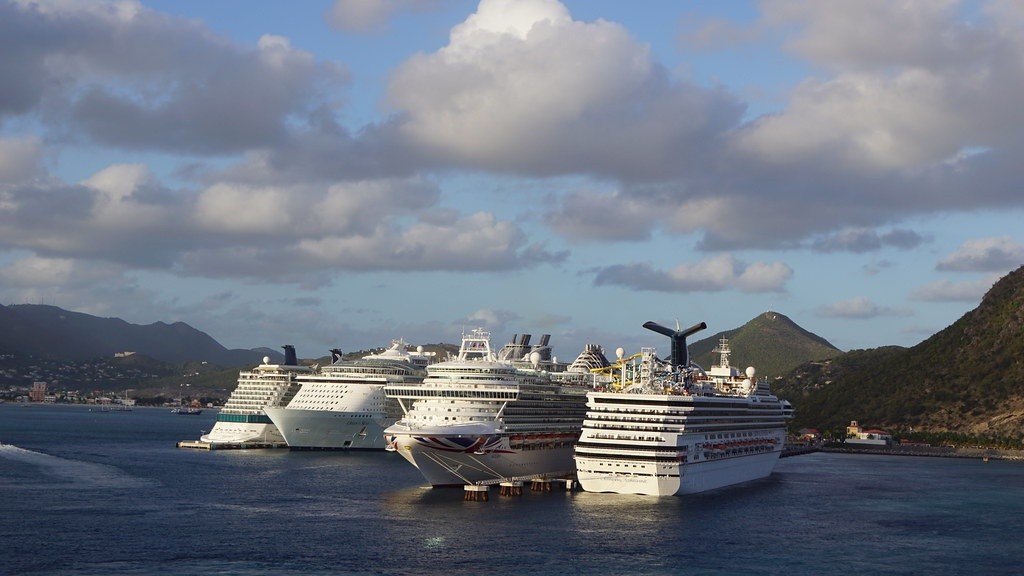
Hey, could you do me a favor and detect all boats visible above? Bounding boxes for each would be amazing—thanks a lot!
[178,410,202,415]
[380,326,619,489]
[573,321,792,498]
[177,344,349,448]
[259,340,457,448]
